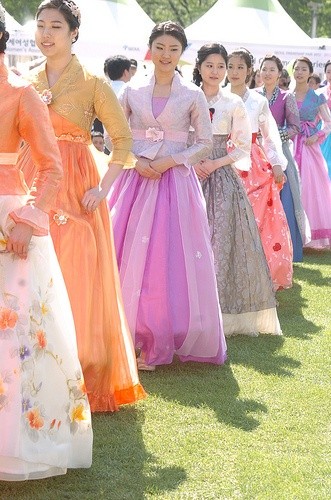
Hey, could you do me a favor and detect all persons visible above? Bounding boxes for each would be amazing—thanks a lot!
[12,0,146,417]
[0,9,93,481]
[9,19,331,372]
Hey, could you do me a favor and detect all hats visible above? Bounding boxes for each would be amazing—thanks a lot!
[130,59,137,68]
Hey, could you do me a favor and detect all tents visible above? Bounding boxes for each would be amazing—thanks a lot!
[31,0,311,43]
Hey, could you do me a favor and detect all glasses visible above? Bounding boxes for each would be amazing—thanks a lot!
[279,81,290,86]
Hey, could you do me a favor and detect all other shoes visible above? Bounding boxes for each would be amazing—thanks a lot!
[137,350,156,372]
